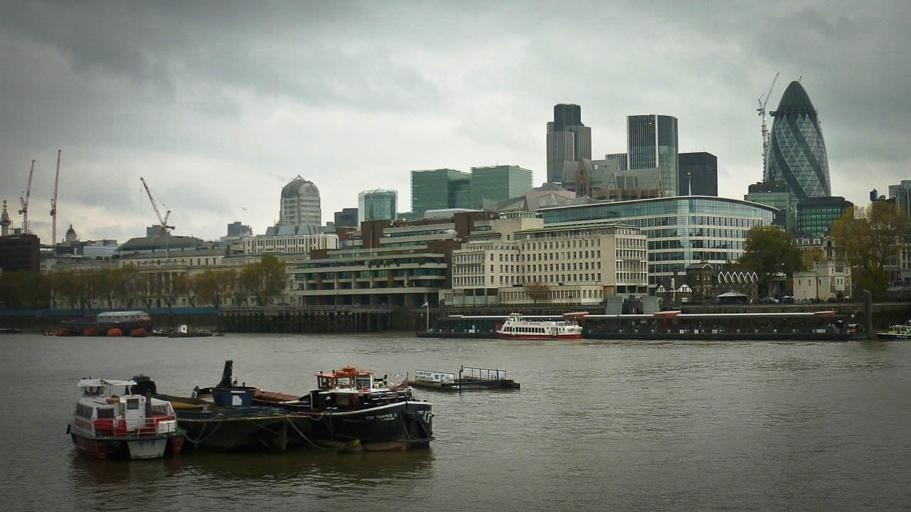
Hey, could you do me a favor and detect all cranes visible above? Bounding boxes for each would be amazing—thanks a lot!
[139,176,175,235]
[757,71,780,183]
[18,148,61,247]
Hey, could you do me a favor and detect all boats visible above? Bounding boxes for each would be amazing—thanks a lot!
[43,307,213,337]
[873,319,911,339]
[495,311,583,340]
[67,376,186,460]
[190,360,437,453]
[125,374,315,456]
[416,306,866,342]
